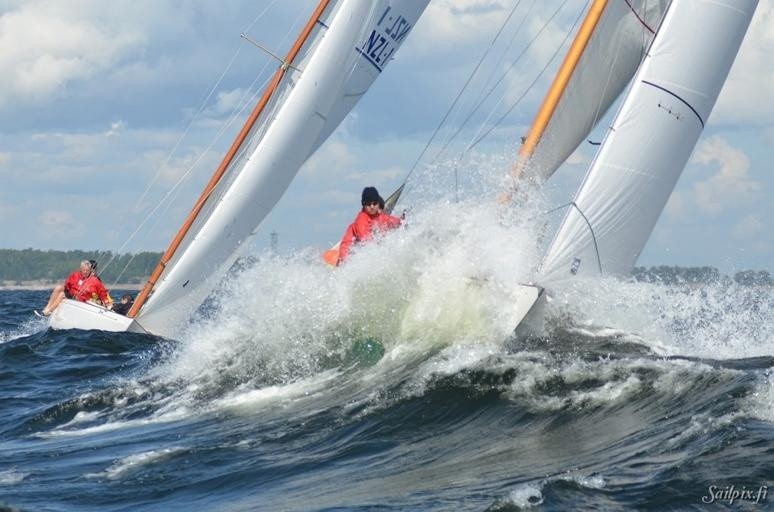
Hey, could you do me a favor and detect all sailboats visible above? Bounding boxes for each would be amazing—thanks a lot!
[331,3,762,349]
[42,3,435,341]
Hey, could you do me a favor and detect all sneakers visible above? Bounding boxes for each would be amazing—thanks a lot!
[34,309,46,317]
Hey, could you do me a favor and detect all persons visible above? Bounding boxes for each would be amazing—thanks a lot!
[108,293,133,316]
[339,187,402,266]
[89,260,100,284]
[31,259,111,317]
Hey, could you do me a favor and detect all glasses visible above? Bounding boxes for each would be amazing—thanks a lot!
[366,202,377,206]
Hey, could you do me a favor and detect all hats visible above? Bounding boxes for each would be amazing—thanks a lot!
[362,186,378,204]
